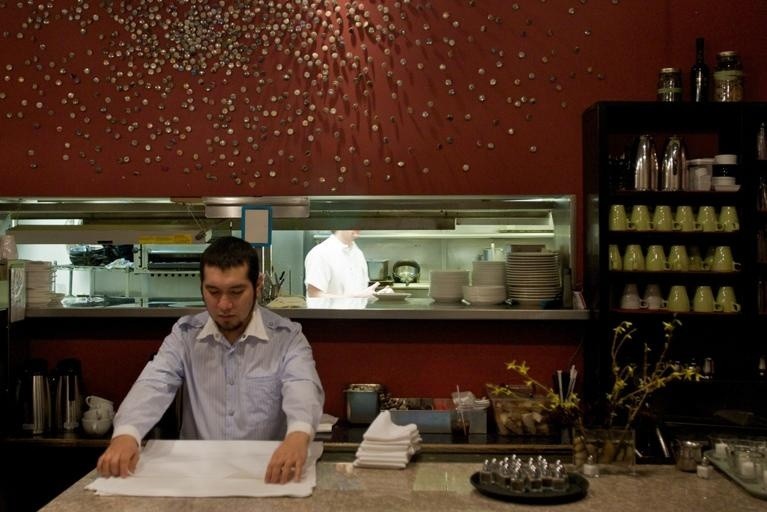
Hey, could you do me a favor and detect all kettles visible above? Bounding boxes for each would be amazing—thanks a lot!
[14,358,52,436]
[53,357,84,431]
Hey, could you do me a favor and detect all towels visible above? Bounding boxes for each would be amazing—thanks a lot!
[350,407,421,470]
[83,434,324,499]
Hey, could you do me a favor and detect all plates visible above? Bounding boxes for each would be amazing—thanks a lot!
[714,185,741,192]
[471,261,505,286]
[709,176,734,186]
[56,293,66,302]
[505,252,560,306]
[461,285,506,306]
[427,270,469,304]
[372,292,412,301]
[713,154,738,166]
[25,262,56,308]
[687,158,713,165]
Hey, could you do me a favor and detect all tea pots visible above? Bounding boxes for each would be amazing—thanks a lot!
[631,134,658,192]
[659,135,687,191]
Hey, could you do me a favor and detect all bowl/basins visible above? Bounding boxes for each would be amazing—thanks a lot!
[82,419,112,435]
[84,406,113,420]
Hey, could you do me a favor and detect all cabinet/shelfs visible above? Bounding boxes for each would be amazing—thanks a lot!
[582,100,764,435]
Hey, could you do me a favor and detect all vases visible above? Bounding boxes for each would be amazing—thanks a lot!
[606,427,637,477]
[560,426,577,445]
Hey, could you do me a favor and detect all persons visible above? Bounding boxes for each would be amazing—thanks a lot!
[304,230,379,308]
[97,236,324,484]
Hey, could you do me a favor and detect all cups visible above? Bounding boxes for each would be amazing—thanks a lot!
[686,166,712,192]
[608,203,637,233]
[608,243,623,272]
[708,428,766,491]
[669,438,703,472]
[665,285,696,312]
[688,245,710,272]
[620,282,649,310]
[710,245,740,272]
[652,205,681,231]
[692,286,723,312]
[606,149,631,190]
[719,206,739,233]
[667,245,690,271]
[642,284,671,311]
[622,244,645,272]
[715,286,740,313]
[644,245,672,271]
[696,205,724,233]
[674,205,704,231]
[630,205,655,231]
[84,394,113,408]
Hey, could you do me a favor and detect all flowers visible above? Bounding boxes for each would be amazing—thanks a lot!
[605,314,714,461]
[486,358,583,437]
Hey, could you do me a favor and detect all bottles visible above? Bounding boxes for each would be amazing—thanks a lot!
[583,455,599,478]
[695,455,714,479]
[479,453,570,492]
[688,37,708,103]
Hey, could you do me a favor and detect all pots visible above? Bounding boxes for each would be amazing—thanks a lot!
[366,258,389,281]
[392,259,421,287]
